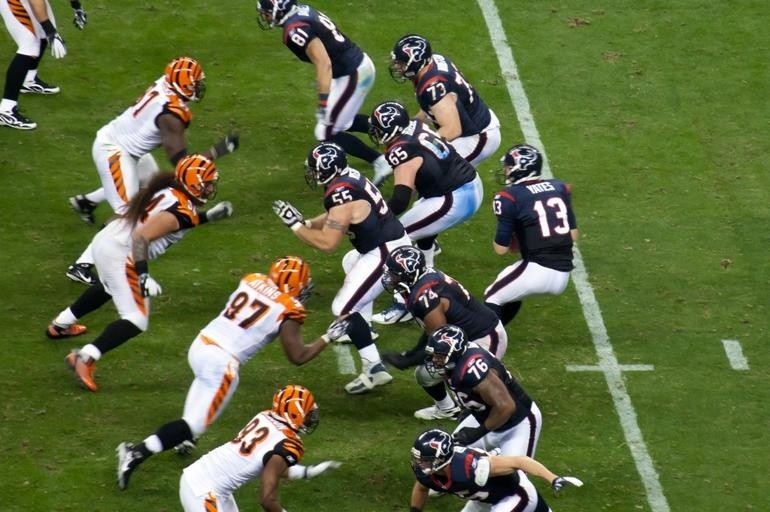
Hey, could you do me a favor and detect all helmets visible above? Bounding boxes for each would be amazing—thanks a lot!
[424,324,466,380]
[388,36,431,82]
[164,56,206,103]
[386,246,427,287]
[271,386,320,435]
[367,101,409,147]
[499,144,541,186]
[411,428,454,476]
[304,143,347,187]
[256,1,298,31]
[173,153,219,204]
[269,256,311,299]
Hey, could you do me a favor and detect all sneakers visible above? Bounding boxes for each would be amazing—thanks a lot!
[69,193,99,224]
[64,264,99,287]
[414,406,461,422]
[0,104,37,131]
[64,350,98,392]
[371,155,394,187]
[46,320,88,338]
[343,362,394,395]
[18,77,60,95]
[371,298,417,325]
[114,440,145,490]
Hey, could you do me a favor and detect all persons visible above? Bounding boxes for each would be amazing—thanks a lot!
[259,1,394,187]
[176,384,342,512]
[371,101,484,325]
[409,429,583,512]
[46,155,237,394]
[483,144,579,327]
[381,246,508,421]
[271,144,411,393]
[62,55,240,286]
[426,327,543,498]
[115,254,349,491]
[388,35,501,268]
[0,0,86,128]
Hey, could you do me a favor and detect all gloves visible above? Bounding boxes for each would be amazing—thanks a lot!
[549,474,584,499]
[68,0,88,31]
[321,318,351,343]
[383,352,409,371]
[205,201,233,224]
[271,199,312,232]
[313,113,327,142]
[137,272,163,299]
[39,19,67,60]
[304,458,344,478]
[208,128,240,160]
